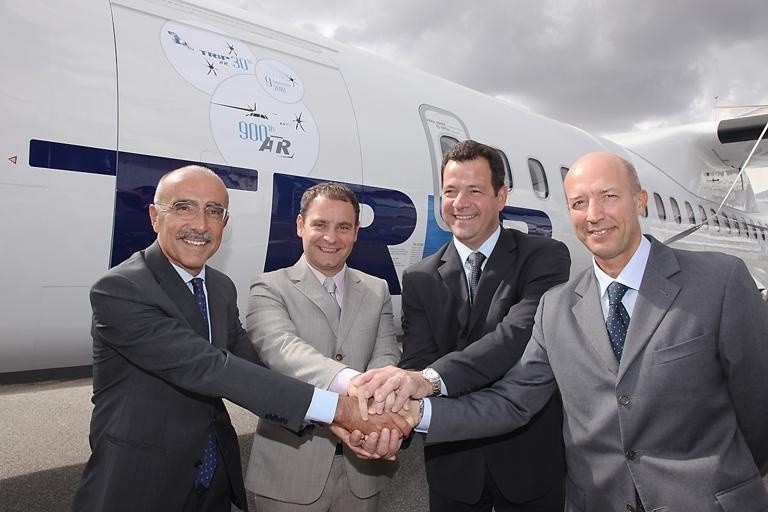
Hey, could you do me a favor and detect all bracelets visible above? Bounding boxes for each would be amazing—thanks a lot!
[414,398,424,427]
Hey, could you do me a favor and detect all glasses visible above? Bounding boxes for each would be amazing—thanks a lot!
[154,199,230,224]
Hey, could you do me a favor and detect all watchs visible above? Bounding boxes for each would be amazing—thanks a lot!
[421,367,441,397]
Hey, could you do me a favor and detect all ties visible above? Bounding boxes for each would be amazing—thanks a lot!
[189,277,220,490]
[324,276,337,311]
[466,250,488,308]
[604,281,631,365]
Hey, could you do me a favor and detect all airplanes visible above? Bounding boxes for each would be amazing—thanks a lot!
[0,0,768,376]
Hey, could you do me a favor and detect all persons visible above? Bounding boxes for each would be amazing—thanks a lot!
[241,180,399,511]
[349,137,573,512]
[68,162,411,510]
[386,147,768,512]
